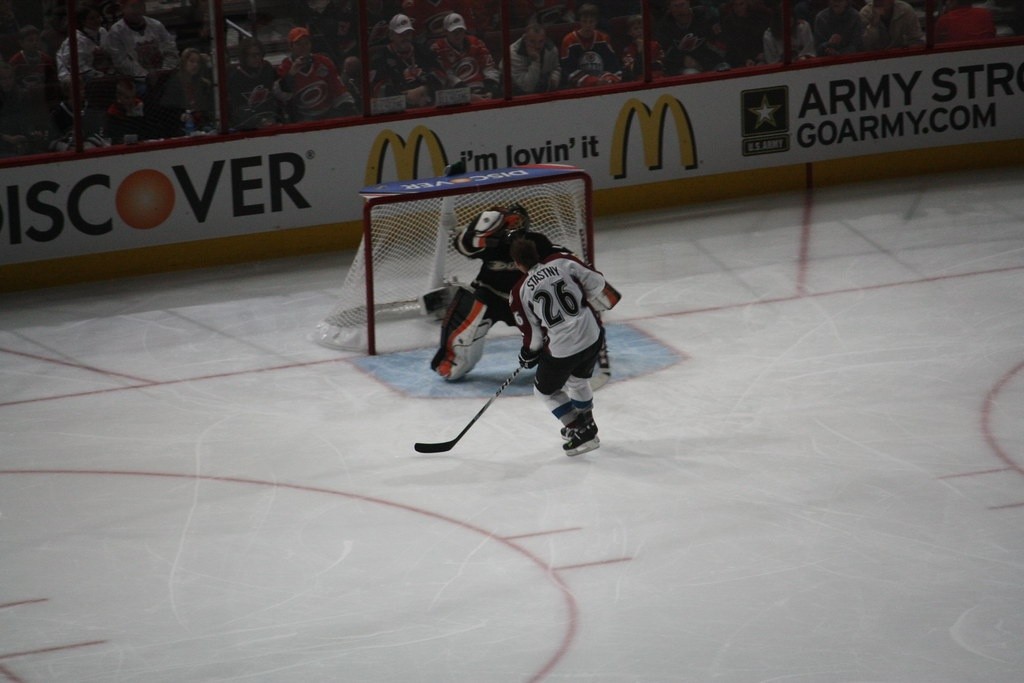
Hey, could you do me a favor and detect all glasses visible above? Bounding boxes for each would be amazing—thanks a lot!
[87,14,101,21]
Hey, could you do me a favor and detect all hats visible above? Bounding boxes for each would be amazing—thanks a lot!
[287,26,311,43]
[389,13,415,34]
[443,13,467,32]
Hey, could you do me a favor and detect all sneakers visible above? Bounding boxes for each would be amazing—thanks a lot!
[561,410,600,457]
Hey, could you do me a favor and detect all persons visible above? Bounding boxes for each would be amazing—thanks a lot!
[430,205,573,381]
[508,238,605,457]
[0,0,996,160]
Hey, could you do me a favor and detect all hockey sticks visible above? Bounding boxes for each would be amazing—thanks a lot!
[414,366,526,453]
[577,227,612,392]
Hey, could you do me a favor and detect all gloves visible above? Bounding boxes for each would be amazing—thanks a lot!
[518,346,543,369]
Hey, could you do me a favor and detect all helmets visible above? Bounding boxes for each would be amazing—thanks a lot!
[504,204,530,239]
[578,51,604,73]
